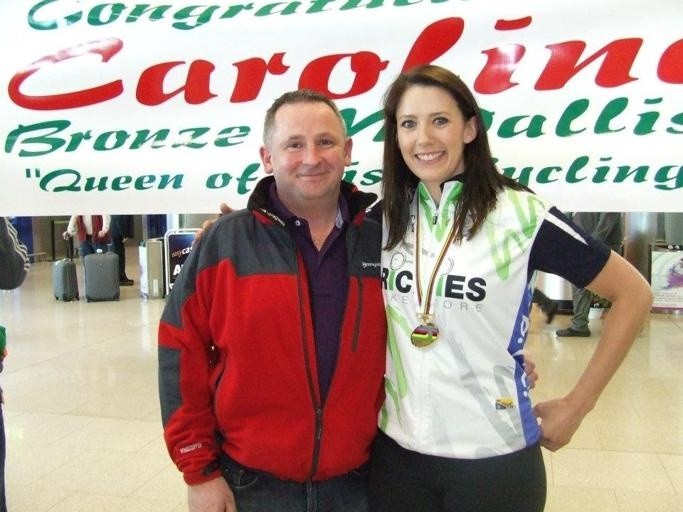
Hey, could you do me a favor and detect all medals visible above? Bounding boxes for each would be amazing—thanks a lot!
[411,322,440,346]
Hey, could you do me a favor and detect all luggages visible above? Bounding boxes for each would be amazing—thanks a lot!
[52,257,79,302]
[83,251,121,302]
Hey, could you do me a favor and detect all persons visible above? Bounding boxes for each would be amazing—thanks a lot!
[156,89,539,512]
[533,288,560,324]
[190,60,655,512]
[0,217,29,512]
[554,212,623,338]
[109,216,135,286]
[61,215,112,257]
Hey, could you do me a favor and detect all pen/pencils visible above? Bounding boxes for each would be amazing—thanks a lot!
[209,346,218,368]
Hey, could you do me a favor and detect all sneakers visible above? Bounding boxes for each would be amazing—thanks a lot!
[547,302,558,323]
[556,328,590,337]
[119,281,133,286]
